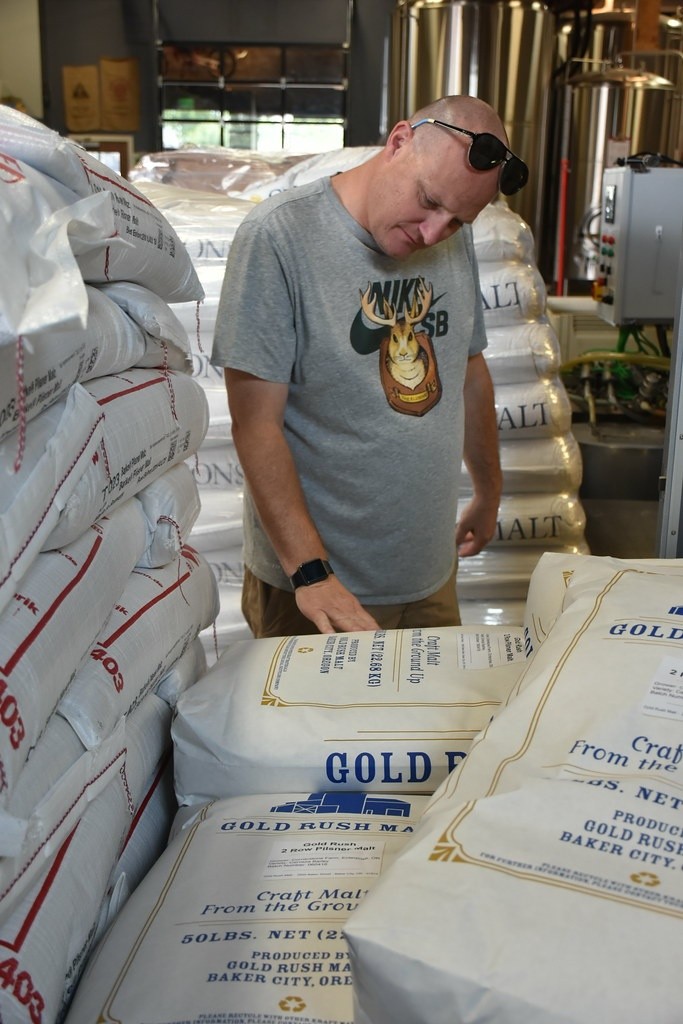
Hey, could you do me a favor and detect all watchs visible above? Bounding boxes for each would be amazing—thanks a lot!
[287,559,333,589]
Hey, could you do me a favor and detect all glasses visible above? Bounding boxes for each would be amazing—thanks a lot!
[411,118,529,196]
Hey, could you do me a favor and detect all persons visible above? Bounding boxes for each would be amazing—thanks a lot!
[213,97,511,635]
